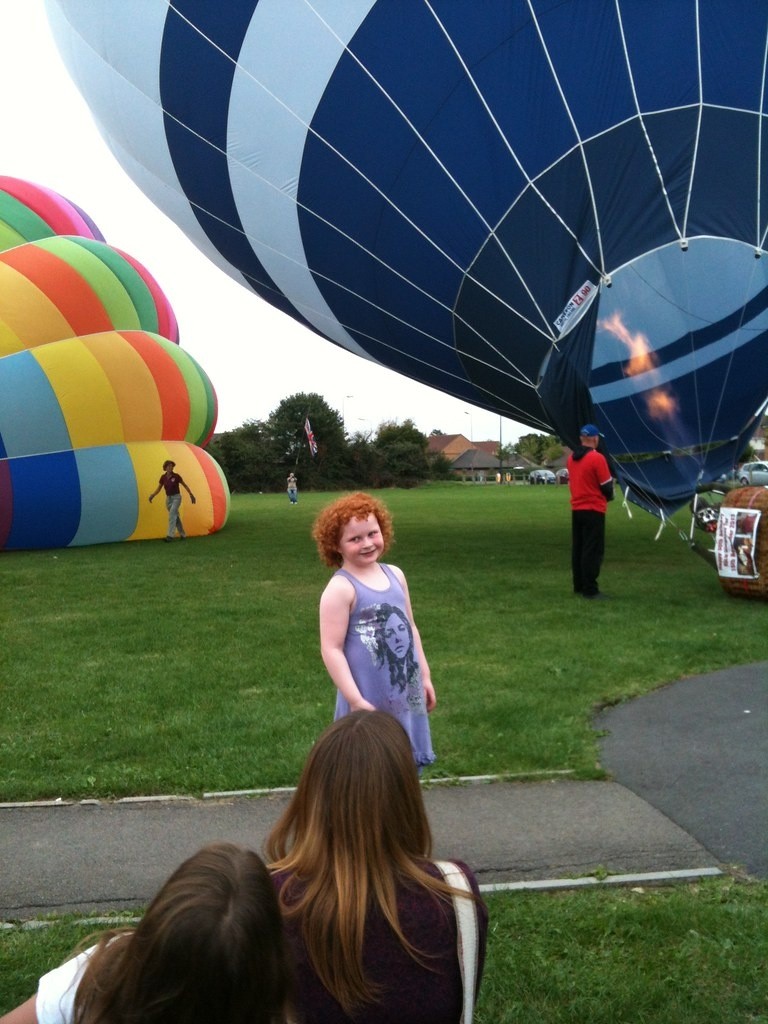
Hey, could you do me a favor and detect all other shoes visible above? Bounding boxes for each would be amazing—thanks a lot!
[163,536,174,543]
[290,501,294,505]
[294,501,297,504]
[575,589,613,600]
[180,535,186,541]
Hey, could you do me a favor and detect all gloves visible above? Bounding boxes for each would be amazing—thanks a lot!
[190,495,196,504]
[149,494,154,503]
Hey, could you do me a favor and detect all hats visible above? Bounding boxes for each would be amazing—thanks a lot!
[163,461,175,471]
[580,424,605,438]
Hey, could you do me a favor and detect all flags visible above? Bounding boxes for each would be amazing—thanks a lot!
[305,418,318,456]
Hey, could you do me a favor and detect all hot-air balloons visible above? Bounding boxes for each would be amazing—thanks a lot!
[45,0,768,599]
[0,175,230,552]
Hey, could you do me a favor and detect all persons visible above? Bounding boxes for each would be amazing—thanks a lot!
[0,843,284,1024]
[262,710,489,1024]
[287,473,297,504]
[313,493,437,773]
[149,461,196,543]
[568,425,614,599]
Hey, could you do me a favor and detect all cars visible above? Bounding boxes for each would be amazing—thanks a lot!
[529,469,556,484]
[555,468,569,484]
[737,460,768,486]
